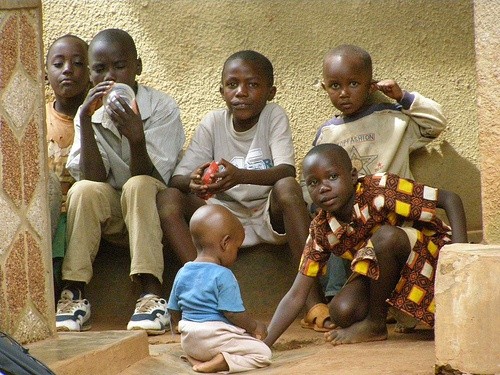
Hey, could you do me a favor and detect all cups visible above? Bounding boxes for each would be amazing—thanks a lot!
[102,81,135,115]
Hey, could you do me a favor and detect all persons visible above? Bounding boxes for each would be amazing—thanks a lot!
[311,44,446,310]
[261,143,468,348]
[45,34,96,309]
[54,29,186,335]
[167,204,273,374]
[156,49,339,334]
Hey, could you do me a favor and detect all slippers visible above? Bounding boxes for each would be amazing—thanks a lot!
[301,303,342,332]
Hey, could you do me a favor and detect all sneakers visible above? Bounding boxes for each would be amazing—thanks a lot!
[55,286,91,331]
[127,294,172,331]
